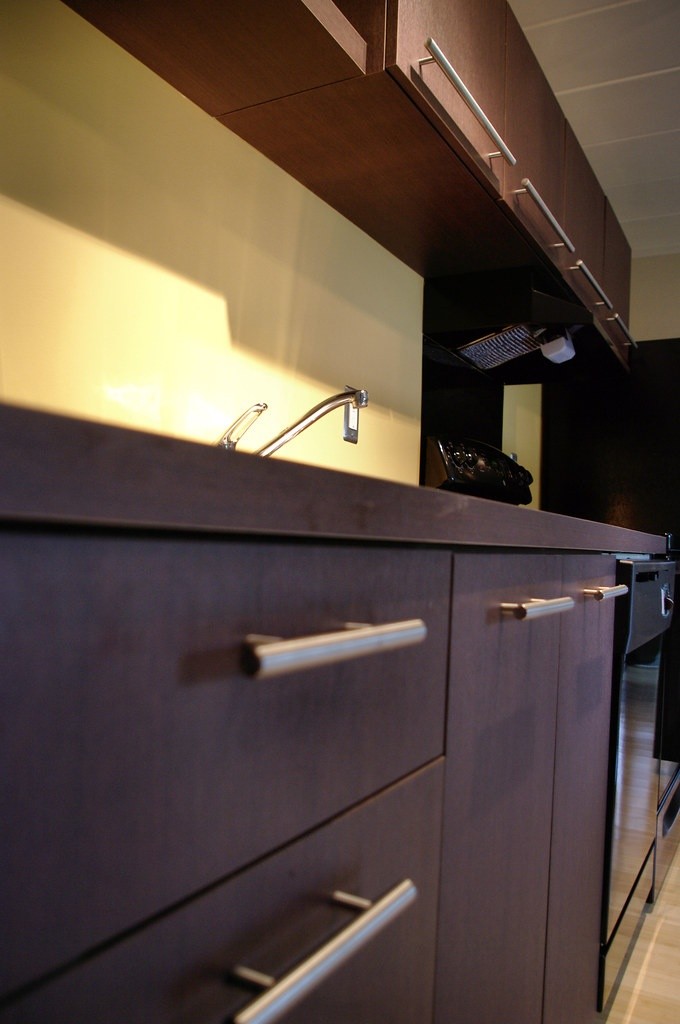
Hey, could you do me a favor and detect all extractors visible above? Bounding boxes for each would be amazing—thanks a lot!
[421,237,593,387]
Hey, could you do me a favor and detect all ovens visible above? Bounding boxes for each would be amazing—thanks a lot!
[598,557,676,948]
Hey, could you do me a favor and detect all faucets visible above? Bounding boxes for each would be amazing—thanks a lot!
[254,388,367,460]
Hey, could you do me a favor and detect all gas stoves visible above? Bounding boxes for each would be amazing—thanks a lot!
[434,436,680,552]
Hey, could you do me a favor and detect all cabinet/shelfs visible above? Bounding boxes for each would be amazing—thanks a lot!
[60,0,639,384]
[0,397,678,1024]
